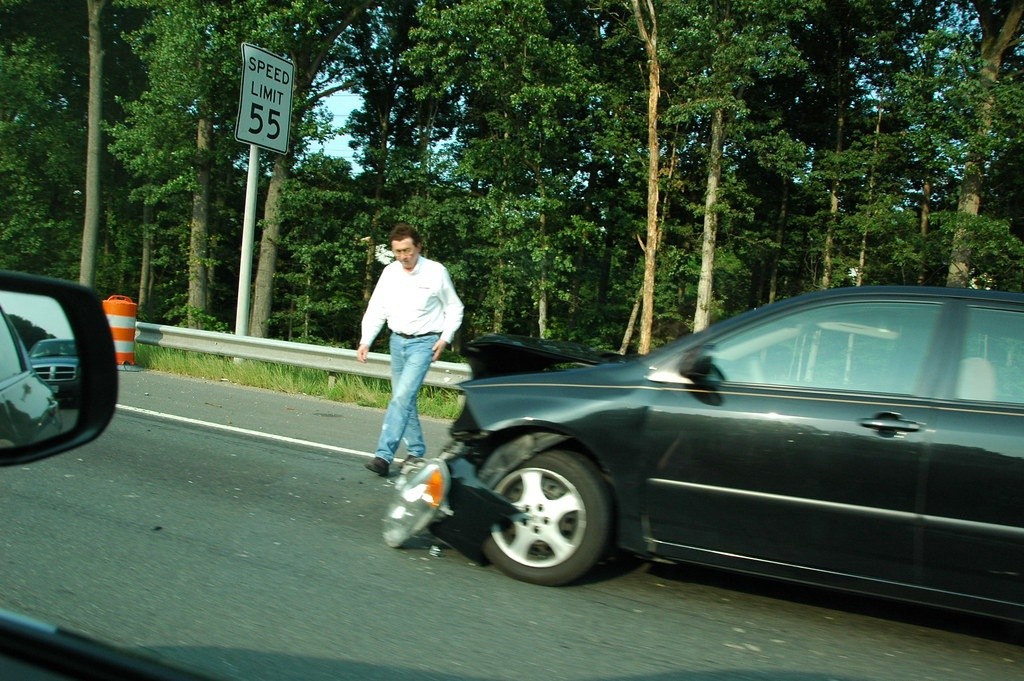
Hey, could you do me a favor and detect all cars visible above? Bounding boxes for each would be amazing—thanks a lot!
[382,282,1024,643]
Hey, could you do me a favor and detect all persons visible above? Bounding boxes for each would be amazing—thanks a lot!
[357,225,464,476]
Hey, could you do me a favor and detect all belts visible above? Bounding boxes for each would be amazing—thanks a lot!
[395,332,435,339]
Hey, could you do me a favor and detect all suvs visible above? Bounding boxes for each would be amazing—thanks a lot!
[26,338,83,409]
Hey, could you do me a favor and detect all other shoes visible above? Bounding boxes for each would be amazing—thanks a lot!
[365,457,390,476]
[400,455,424,468]
